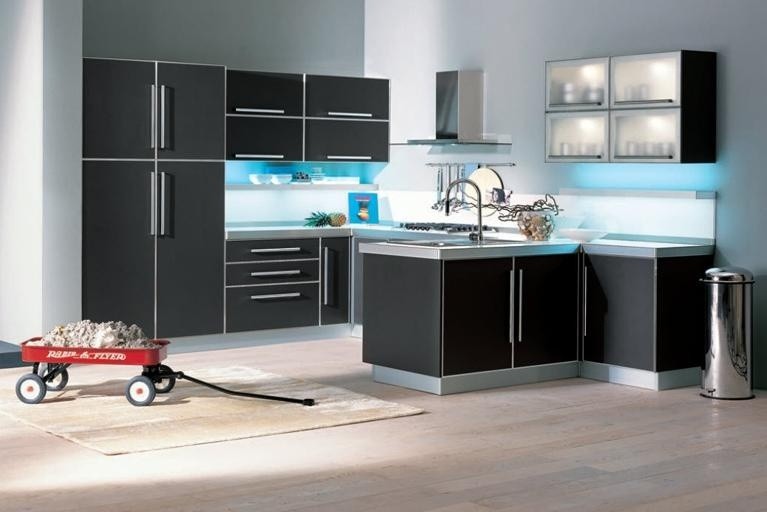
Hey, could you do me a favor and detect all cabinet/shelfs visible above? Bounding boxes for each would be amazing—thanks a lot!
[227,231,352,332]
[82,54,226,162]
[580,248,706,392]
[357,250,579,383]
[82,161,224,344]
[543,48,716,167]
[224,69,391,166]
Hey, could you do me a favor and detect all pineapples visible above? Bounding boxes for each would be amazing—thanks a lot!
[303,210,346,227]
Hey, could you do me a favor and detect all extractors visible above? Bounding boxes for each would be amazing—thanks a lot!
[387,68,513,148]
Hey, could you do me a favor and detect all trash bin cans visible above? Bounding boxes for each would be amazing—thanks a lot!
[699,266,756,400]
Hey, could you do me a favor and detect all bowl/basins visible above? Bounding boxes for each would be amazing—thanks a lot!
[270,174,293,185]
[248,173,271,185]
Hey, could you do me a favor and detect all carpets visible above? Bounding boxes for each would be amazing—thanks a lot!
[3,364,426,456]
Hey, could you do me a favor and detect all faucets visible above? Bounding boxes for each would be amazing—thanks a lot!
[445,178,483,240]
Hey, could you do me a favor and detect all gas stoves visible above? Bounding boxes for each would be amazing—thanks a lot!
[399,221,492,234]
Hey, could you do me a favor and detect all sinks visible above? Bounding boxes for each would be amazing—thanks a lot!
[381,240,465,250]
